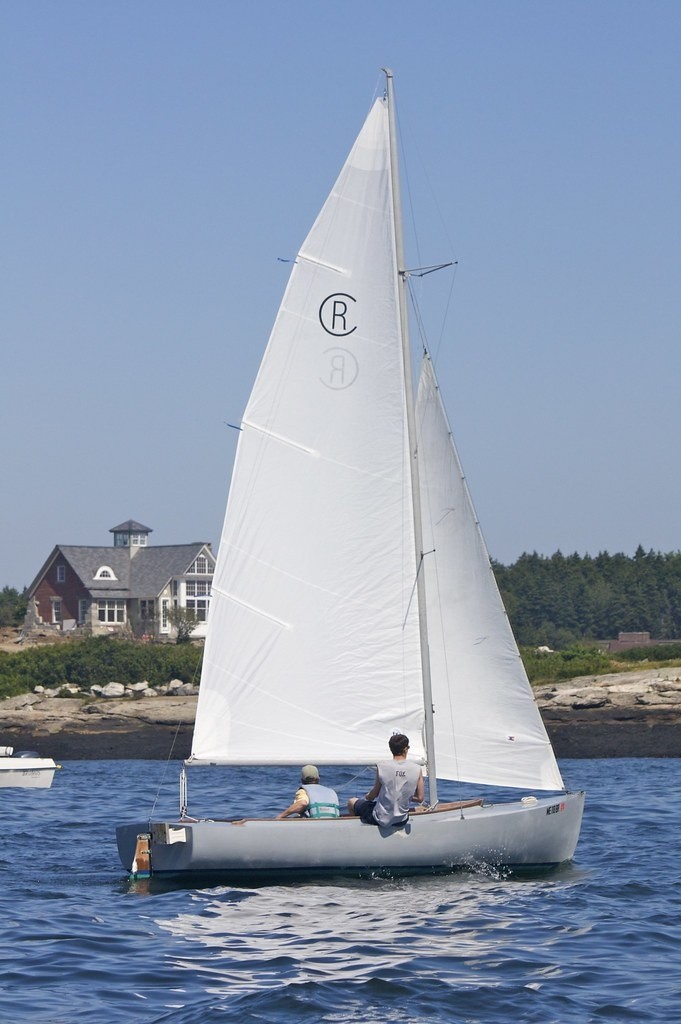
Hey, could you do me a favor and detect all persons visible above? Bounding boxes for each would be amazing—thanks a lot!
[347,733,425,828]
[277,764,340,819]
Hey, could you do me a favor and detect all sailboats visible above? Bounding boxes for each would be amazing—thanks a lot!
[115,67,586,898]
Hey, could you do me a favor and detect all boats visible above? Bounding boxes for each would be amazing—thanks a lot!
[0,746,62,789]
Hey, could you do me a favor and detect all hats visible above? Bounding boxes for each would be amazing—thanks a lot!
[302,765,319,779]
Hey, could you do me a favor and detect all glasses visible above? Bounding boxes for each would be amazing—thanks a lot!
[406,745,410,749]
